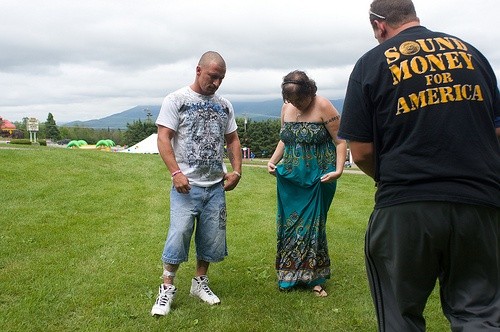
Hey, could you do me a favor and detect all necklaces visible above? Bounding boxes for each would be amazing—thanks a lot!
[295,109,307,117]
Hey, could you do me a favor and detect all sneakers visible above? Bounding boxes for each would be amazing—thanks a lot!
[151,283,177,316]
[189,275,221,306]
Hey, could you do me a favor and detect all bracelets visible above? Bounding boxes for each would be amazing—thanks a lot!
[172,170,181,177]
[234,171,242,178]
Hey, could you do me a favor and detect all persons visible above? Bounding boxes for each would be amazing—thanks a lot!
[337,0,500,332]
[149,51,242,317]
[268,69,347,297]
[345,161,351,168]
[250,152,255,161]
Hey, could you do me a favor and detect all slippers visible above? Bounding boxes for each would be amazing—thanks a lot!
[311,287,324,294]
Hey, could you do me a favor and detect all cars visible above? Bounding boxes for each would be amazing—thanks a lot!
[57,139,70,145]
